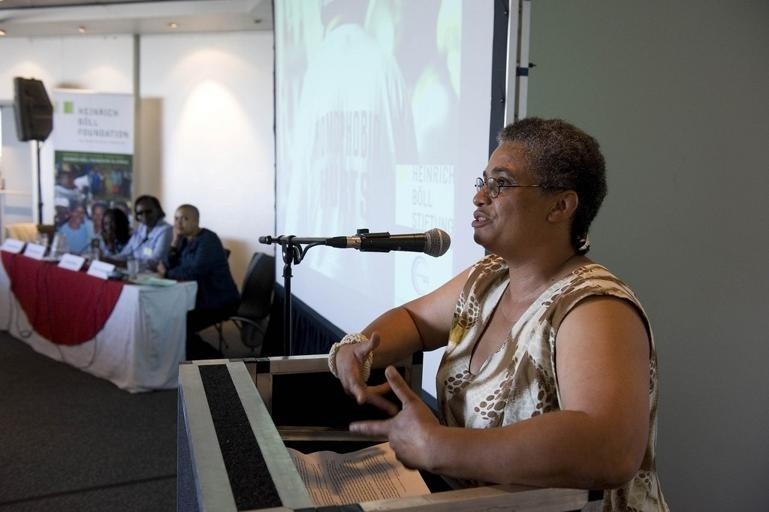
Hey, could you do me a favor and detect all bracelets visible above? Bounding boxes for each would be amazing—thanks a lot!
[327,332,374,383]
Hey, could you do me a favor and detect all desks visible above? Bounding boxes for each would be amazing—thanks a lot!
[0,250,197,394]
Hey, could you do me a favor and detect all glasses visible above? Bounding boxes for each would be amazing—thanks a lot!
[475,177,547,198]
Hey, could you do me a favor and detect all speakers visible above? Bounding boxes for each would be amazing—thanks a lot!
[14,76,52,142]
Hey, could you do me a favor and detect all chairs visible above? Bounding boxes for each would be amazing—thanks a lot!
[224,249,231,258]
[218,252,276,359]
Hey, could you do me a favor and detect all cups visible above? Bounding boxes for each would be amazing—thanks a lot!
[127,258,141,280]
[35,231,48,248]
[87,247,98,265]
[54,230,67,256]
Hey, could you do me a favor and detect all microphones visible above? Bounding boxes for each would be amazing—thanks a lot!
[329,228,450,258]
[108,237,149,280]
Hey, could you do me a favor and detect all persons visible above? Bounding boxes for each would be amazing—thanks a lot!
[94,195,242,360]
[327,118,671,512]
[54,163,131,253]
